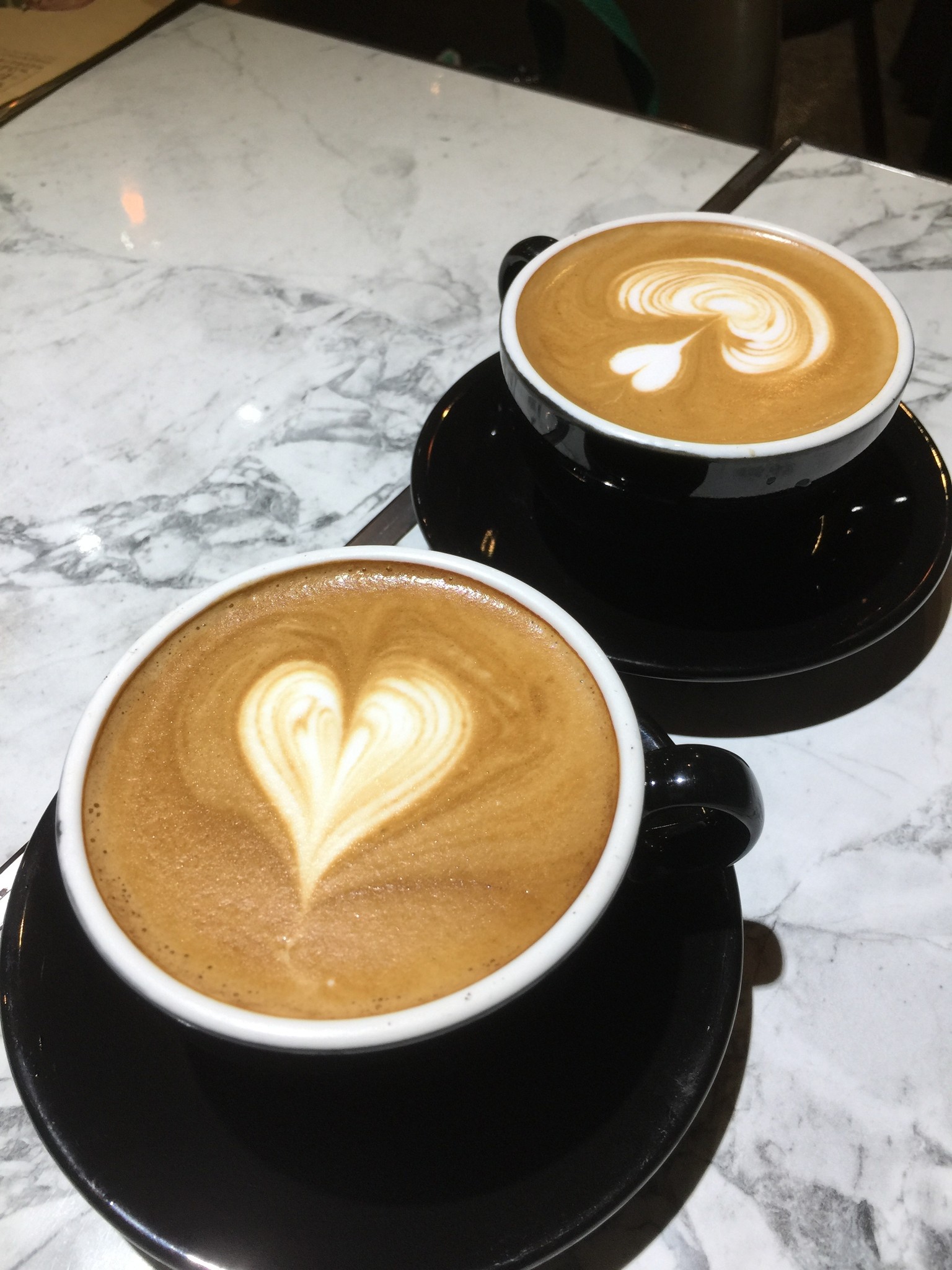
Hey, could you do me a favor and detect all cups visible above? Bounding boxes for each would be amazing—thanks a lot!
[496,211,918,511]
[57,547,763,1054]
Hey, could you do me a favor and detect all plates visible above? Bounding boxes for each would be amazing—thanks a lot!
[410,354,952,681]
[0,722,747,1270]
